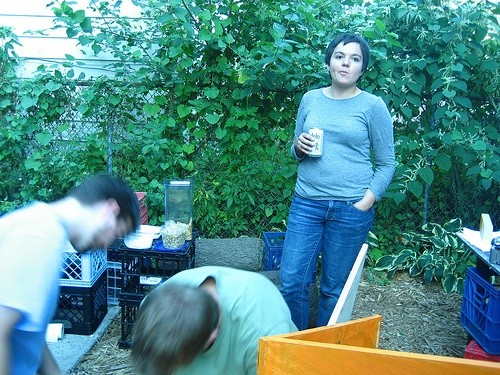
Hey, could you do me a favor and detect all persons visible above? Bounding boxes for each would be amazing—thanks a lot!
[278,33,396,332]
[0,176,139,375]
[131,266,299,375]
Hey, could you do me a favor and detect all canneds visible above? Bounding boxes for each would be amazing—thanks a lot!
[308,129,323,157]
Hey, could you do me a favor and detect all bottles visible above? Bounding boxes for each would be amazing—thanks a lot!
[489,238,500,265]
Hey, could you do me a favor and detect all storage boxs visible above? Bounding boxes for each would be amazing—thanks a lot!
[49,192,196,350]
[459,267,500,363]
[262,232,287,271]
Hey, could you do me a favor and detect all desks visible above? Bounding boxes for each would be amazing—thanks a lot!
[455,231,500,322]
